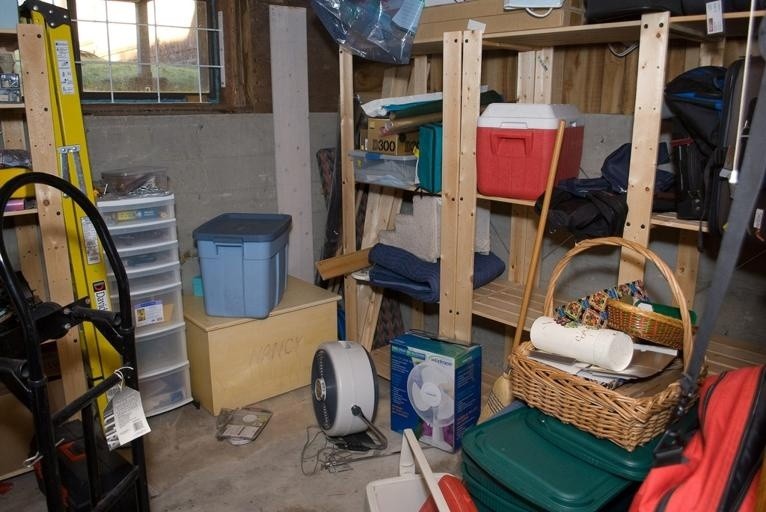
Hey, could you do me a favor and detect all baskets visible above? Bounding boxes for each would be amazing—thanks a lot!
[509,236,709,453]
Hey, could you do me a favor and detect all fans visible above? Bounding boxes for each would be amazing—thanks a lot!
[311,341,386,451]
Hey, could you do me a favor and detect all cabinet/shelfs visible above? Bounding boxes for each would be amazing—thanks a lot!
[0,24,87,479]
[99,193,194,417]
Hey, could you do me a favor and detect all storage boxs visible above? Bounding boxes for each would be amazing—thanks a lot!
[367,117,419,155]
[478,102,585,200]
[185,274,343,416]
[419,0,584,23]
[388,329,481,453]
[460,450,641,510]
[193,212,291,318]
[412,10,583,41]
[348,148,416,188]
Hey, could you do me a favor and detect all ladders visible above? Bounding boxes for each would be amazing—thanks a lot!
[15,0,132,440]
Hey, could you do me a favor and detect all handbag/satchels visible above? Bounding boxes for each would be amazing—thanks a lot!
[628,366,766,512]
